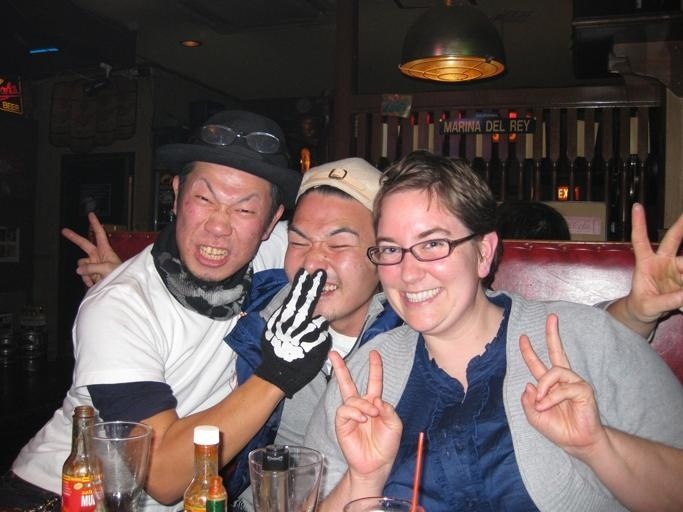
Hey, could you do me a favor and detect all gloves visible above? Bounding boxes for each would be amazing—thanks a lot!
[254,267,332,399]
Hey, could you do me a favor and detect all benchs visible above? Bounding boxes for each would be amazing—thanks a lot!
[90,226,683,384]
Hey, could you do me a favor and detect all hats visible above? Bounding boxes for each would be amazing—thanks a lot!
[294,157,388,211]
[151,110,302,192]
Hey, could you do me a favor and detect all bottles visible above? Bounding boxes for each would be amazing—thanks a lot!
[60,403,292,512]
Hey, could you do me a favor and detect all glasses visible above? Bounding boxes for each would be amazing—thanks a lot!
[366,232,485,266]
[186,124,294,163]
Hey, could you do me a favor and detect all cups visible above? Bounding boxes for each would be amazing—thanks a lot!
[341,496,423,511]
[247,444,324,512]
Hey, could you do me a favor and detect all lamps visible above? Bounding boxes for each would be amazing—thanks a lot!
[397,0,507,82]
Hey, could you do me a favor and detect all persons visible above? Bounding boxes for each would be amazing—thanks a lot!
[496,195,572,242]
[294,146,683,512]
[59,155,683,511]
[6,109,333,511]
